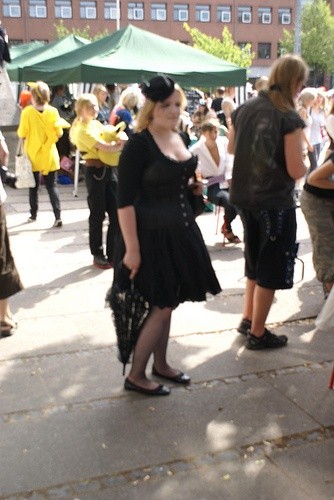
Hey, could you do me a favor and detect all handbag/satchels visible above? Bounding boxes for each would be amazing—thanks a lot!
[14,139,36,189]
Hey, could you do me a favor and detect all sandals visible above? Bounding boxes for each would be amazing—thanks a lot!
[221,225,240,242]
[0,321,18,338]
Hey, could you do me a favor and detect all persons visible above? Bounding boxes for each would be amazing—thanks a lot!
[0,20,8,67]
[300,152,334,300]
[189,120,241,243]
[94,83,141,135]
[71,93,128,268]
[51,85,75,121]
[296,86,334,172]
[116,75,223,394]
[192,86,235,129]
[17,81,63,227]
[0,130,24,334]
[227,55,311,349]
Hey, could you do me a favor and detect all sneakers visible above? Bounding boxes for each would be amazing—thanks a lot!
[237,319,252,335]
[246,328,287,349]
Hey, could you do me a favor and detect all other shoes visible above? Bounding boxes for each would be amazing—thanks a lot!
[55,219,62,227]
[29,215,36,220]
[94,255,112,268]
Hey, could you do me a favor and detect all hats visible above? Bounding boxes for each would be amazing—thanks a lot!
[140,75,174,102]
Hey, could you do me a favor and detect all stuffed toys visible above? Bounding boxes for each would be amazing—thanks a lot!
[98,121,126,166]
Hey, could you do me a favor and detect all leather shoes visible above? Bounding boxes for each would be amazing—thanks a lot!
[152,364,190,383]
[124,377,170,395]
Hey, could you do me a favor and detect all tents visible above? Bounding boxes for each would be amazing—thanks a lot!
[18,24,247,197]
[6,34,92,98]
[8,40,45,59]
